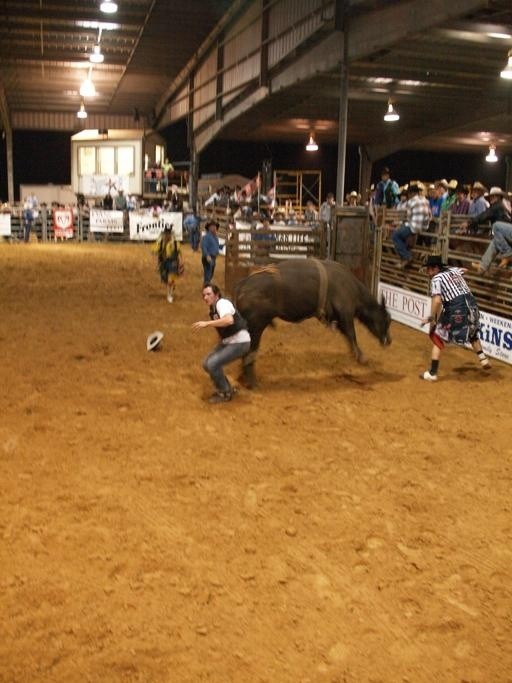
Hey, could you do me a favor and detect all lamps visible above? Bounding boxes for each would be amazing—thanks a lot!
[482,139,499,163]
[382,95,401,123]
[497,46,512,83]
[302,126,320,152]
[72,0,120,120]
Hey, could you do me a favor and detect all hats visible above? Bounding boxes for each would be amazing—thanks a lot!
[378,166,392,174]
[346,191,362,203]
[422,255,448,268]
[470,181,486,190]
[484,185,507,199]
[404,178,470,194]
[146,330,164,352]
[205,219,220,231]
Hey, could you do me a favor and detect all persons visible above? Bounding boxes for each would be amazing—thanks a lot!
[151,228,185,304]
[19,156,201,245]
[198,219,227,287]
[347,166,512,275]
[205,173,337,226]
[189,282,251,401]
[248,215,275,274]
[422,253,492,377]
[181,205,214,250]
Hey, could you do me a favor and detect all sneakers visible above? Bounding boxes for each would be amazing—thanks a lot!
[479,357,492,371]
[207,387,235,404]
[418,370,439,382]
[499,255,512,268]
[167,294,174,304]
[472,262,487,273]
[395,256,413,269]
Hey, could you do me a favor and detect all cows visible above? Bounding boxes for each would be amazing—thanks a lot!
[232,258,392,391]
